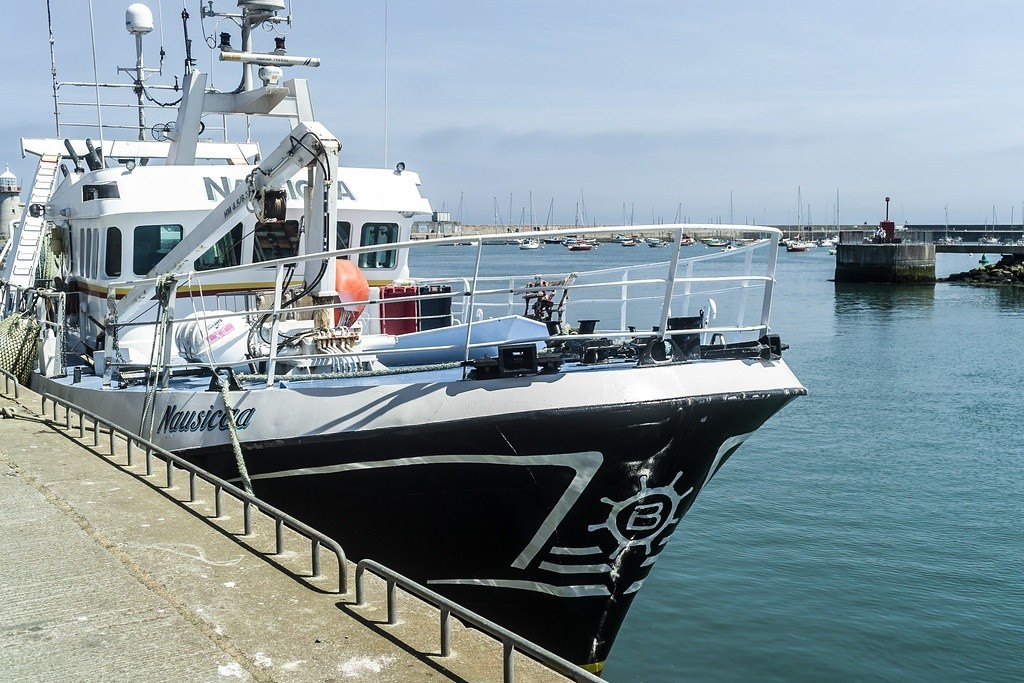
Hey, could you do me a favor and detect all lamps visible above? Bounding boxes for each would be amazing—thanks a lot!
[126,158,136,172]
[28,202,46,218]
[396,160,407,172]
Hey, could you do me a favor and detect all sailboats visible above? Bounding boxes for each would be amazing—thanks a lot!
[938,202,1024,245]
[435,185,843,255]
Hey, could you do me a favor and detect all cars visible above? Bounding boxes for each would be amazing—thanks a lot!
[895,225,909,231]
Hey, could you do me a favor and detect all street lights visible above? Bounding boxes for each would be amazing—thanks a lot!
[885,197,890,220]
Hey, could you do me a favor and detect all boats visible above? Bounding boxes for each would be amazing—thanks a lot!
[0,0,807,683]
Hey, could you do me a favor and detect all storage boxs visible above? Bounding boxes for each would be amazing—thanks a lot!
[380,286,417,335]
[418,284,454,331]
